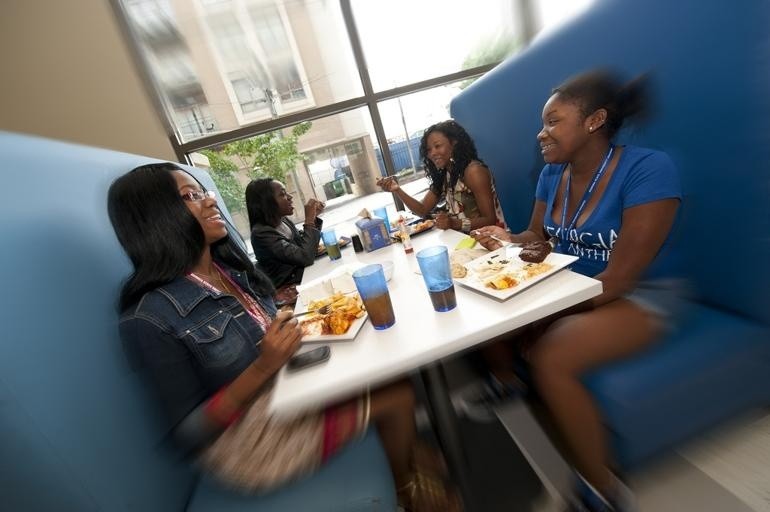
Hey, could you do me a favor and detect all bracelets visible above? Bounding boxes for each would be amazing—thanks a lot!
[461,217,472,233]
[302,221,317,228]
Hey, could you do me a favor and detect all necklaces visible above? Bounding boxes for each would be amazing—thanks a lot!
[190,265,232,294]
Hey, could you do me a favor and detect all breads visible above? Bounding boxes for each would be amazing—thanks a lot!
[519,239,553,263]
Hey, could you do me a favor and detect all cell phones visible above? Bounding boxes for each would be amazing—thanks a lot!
[286,345,330,371]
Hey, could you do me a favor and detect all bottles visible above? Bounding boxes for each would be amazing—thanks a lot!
[350,233,363,253]
[397,218,413,255]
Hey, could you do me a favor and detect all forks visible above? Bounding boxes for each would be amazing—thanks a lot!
[282,296,331,321]
[473,229,523,249]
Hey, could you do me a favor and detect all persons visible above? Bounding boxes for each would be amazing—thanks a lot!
[452,64,698,510]
[245,178,325,310]
[374,118,515,243]
[104,161,466,511]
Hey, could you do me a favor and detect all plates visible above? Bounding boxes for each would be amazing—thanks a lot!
[391,219,434,241]
[314,234,352,257]
[299,291,370,342]
[452,243,580,302]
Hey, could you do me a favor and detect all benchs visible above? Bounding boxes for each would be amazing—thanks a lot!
[0,0,770,512]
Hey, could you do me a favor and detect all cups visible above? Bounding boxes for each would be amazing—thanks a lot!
[414,245,458,312]
[320,228,342,261]
[372,206,391,234]
[351,263,396,331]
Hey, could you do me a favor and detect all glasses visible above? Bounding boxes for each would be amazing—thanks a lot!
[182,189,215,203]
